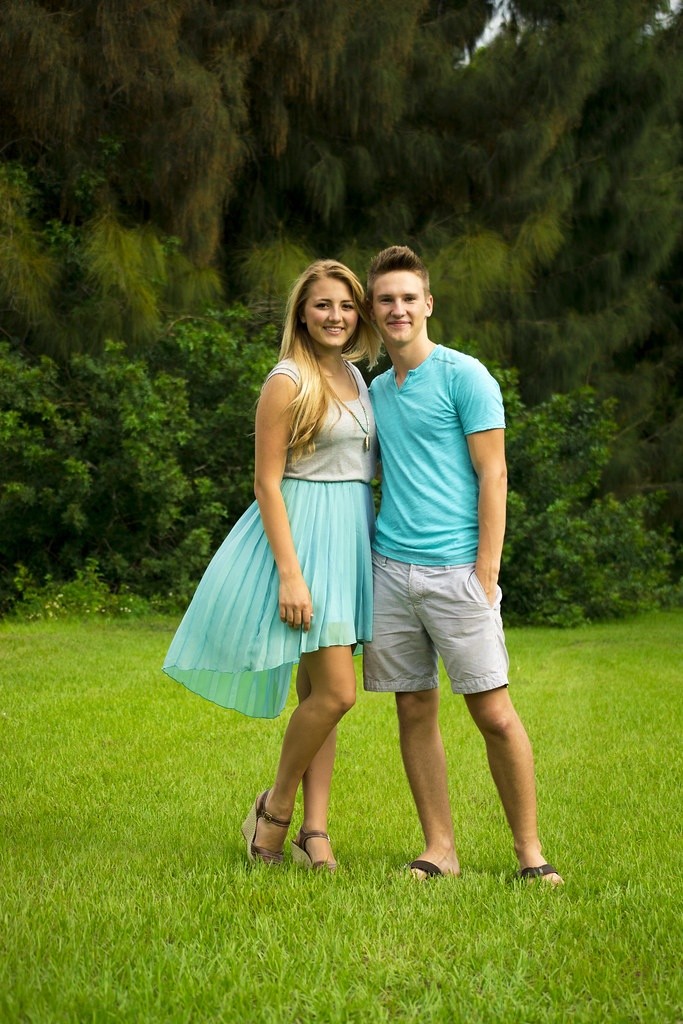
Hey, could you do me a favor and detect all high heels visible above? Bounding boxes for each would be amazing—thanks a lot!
[291,826,338,869]
[242,789,291,866]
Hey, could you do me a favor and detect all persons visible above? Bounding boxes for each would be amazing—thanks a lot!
[367,244,564,889]
[159,258,378,875]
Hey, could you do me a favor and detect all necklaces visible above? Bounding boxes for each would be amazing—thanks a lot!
[320,365,371,451]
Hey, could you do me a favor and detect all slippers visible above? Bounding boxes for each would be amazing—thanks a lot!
[521,864,558,880]
[409,859,441,876]
